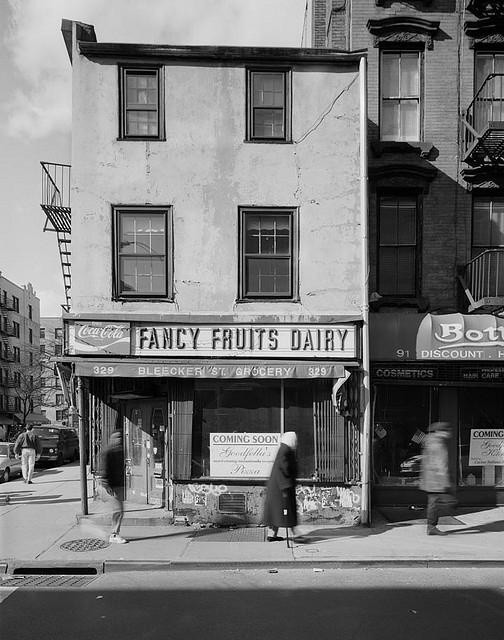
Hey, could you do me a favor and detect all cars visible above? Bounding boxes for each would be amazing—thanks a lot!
[0,442,22,483]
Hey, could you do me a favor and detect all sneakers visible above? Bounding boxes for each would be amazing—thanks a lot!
[267,533,310,542]
[109,533,128,544]
[426,526,449,535]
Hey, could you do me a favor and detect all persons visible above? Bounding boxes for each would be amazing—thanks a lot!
[12,422,41,484]
[260,431,307,543]
[97,431,127,544]
[420,421,456,539]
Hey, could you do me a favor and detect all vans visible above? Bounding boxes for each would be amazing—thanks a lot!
[33,424,79,467]
